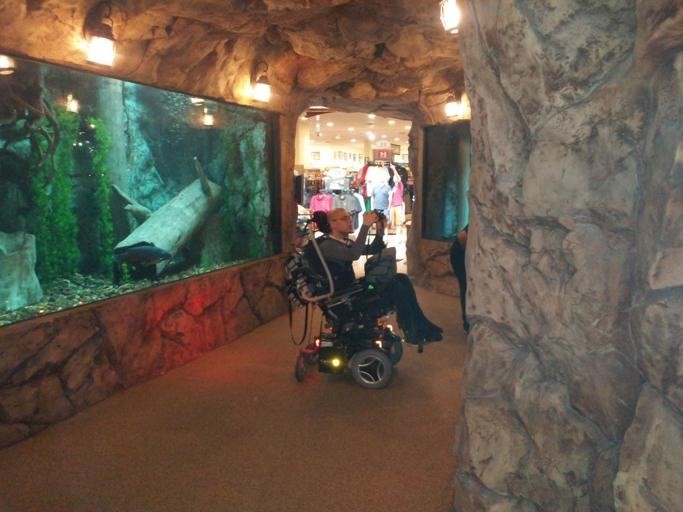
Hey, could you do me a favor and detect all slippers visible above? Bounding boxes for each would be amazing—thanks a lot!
[281,253,328,309]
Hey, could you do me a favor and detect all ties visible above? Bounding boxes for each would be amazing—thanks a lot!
[296,174,304,205]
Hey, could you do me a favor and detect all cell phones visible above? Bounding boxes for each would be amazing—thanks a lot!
[371,209,385,221]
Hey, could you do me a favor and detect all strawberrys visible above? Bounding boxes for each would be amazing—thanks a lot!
[282,209,403,390]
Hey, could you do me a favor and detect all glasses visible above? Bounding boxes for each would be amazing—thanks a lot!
[251,61,272,103]
[443,91,462,120]
[83,0,117,67]
[439,0,463,34]
[199,101,219,126]
[0,54,17,75]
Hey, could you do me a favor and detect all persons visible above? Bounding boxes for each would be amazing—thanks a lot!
[313,207,444,343]
[448,223,470,334]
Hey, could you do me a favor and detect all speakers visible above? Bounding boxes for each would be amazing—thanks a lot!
[410,288,443,334]
[396,292,442,342]
